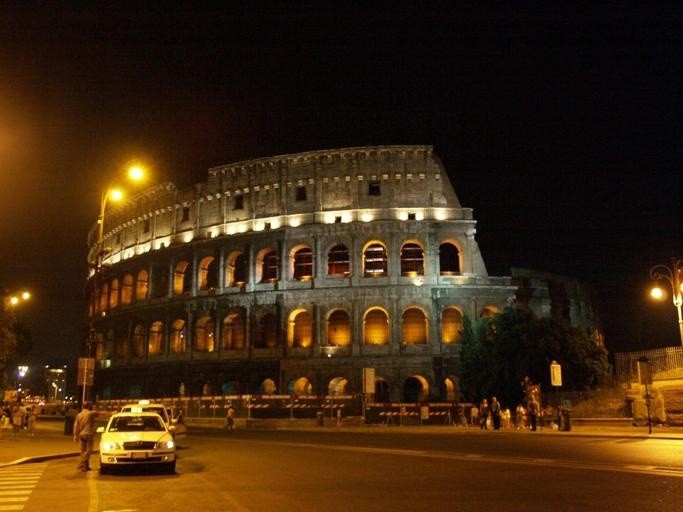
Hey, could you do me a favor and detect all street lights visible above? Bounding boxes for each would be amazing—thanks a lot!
[82,158,149,403]
[649,257,683,344]
[18,365,28,398]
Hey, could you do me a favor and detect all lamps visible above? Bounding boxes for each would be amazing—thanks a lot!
[412,276,424,287]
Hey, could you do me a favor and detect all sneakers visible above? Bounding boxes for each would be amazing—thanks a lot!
[76,463,91,472]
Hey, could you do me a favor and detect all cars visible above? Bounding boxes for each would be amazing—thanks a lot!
[96,403,186,473]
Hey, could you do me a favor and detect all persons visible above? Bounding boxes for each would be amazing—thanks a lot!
[224,404,236,431]
[71,400,117,473]
[336,405,344,428]
[0,393,42,439]
[176,404,184,424]
[447,394,572,432]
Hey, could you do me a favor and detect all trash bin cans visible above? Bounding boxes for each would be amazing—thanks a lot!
[64,416,75,435]
[559,409,571,431]
[316,412,324,426]
